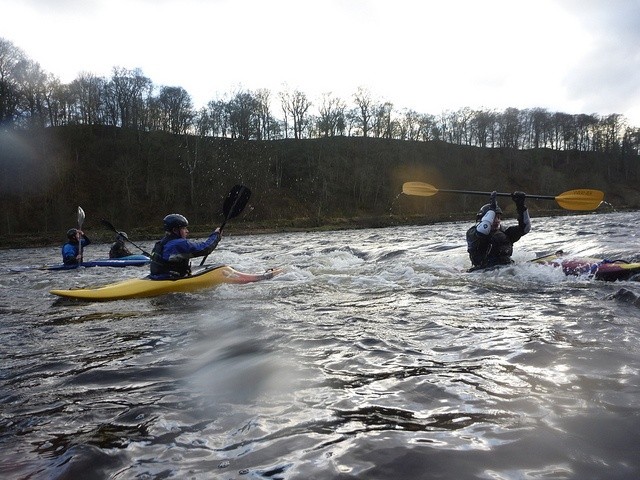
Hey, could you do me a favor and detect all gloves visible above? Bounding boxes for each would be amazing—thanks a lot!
[511,190,527,210]
[488,190,497,210]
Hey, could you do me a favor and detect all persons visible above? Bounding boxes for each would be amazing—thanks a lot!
[466,190,532,269]
[150,213,223,279]
[62,228,93,266]
[109,231,133,258]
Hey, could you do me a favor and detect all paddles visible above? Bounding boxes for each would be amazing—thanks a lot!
[78,206,85,268]
[402,181,604,211]
[96,219,152,258]
[200,185,251,267]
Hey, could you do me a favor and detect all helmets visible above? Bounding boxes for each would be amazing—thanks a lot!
[163,213,189,232]
[476,203,503,221]
[66,228,78,240]
[116,231,128,241]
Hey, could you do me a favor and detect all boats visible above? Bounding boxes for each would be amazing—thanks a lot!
[48,263,282,301]
[526,250,640,283]
[7,253,152,272]
